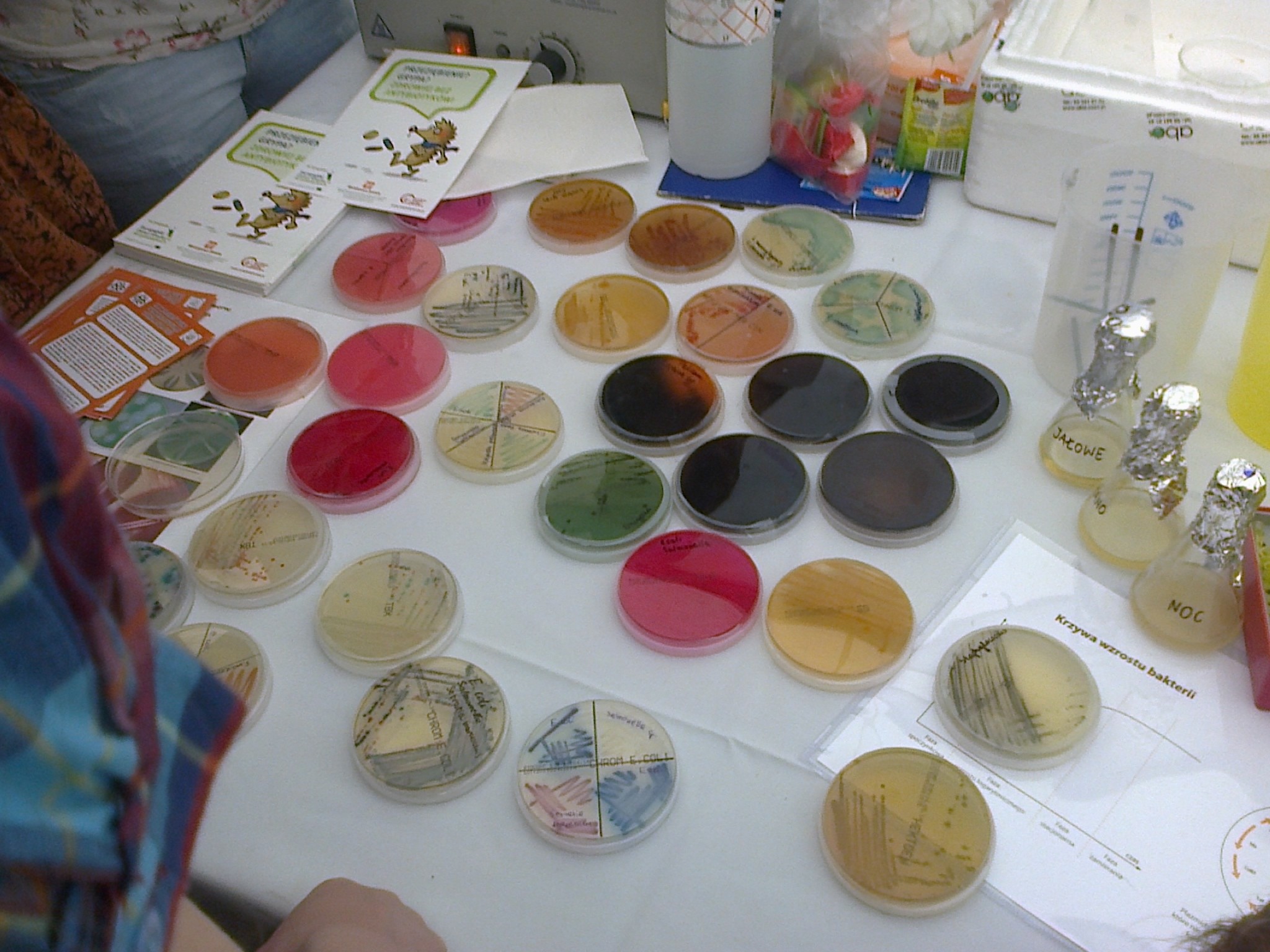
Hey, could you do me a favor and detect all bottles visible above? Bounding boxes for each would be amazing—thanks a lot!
[1130,457,1266,656]
[1079,385,1202,571]
[665,0,775,179]
[1040,305,1154,489]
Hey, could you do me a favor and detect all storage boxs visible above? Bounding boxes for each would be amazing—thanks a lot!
[962,1,1270,270]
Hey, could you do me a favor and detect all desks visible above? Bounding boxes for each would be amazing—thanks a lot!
[19,37,1270,952]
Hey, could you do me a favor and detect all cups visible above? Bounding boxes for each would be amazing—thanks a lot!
[1039,145,1235,416]
[1178,36,1270,93]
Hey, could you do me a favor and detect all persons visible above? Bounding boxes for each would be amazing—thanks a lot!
[0,0,362,234]
[0,0,448,952]
